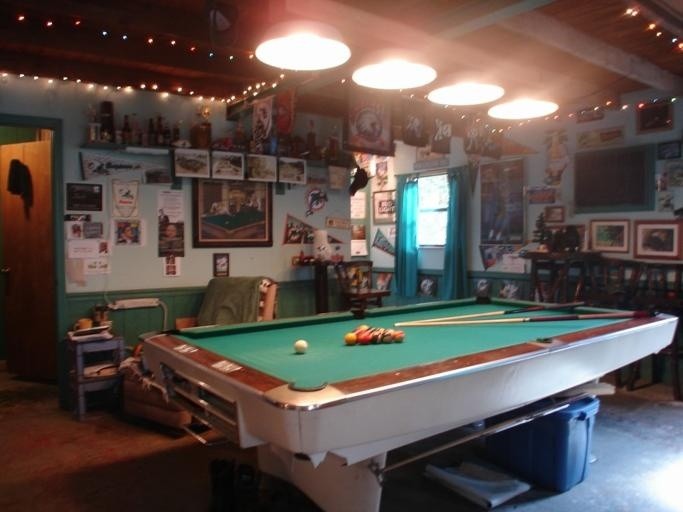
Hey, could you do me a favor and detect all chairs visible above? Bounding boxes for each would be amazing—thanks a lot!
[334,260,391,309]
[519,250,683,404]
[122,277,282,432]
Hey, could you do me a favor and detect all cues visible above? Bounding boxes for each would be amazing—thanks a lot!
[395,310,655,326]
[375,394,588,477]
[395,297,588,325]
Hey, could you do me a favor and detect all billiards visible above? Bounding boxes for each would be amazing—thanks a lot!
[353,324,405,344]
[295,340,307,353]
[345,332,356,345]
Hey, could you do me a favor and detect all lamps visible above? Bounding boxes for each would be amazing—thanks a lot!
[428,69,505,107]
[254,19,350,72]
[351,49,436,92]
[485,86,559,120]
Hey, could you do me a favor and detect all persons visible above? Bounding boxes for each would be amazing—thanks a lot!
[117,225,140,244]
[648,230,666,249]
[160,223,182,249]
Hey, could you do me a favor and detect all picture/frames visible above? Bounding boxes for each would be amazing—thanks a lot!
[212,253,229,277]
[193,177,273,247]
[633,219,682,261]
[543,206,566,223]
[588,219,631,253]
[636,100,674,136]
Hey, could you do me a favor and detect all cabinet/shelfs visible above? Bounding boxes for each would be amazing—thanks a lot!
[65,335,124,421]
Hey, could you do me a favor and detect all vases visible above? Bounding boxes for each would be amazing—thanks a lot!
[311,229,328,263]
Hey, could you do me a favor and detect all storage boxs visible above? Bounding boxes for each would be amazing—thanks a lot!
[476,395,601,494]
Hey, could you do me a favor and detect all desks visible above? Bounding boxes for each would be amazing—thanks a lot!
[290,256,342,313]
[141,296,678,511]
